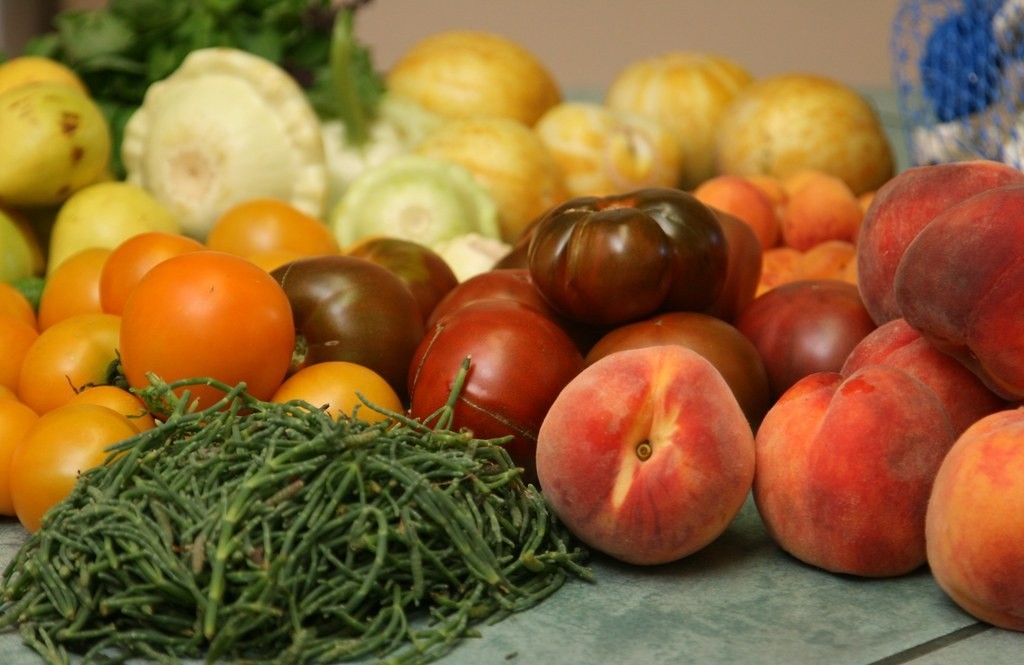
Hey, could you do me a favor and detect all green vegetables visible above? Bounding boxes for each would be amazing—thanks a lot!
[0,0,392,130]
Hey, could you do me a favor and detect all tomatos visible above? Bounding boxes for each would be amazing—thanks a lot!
[0,36,892,539]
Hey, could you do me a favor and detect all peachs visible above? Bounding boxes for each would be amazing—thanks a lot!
[531,156,1024,636]
